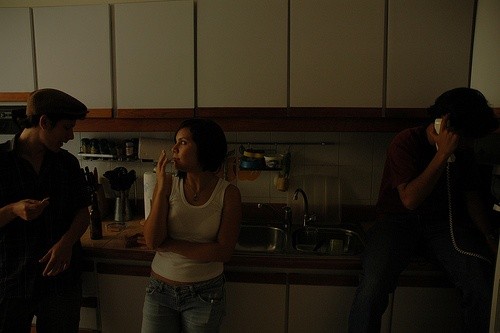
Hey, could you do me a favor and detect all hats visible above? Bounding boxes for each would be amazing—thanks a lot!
[25,88,88,120]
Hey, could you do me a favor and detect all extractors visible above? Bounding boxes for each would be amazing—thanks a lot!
[0,106,28,134]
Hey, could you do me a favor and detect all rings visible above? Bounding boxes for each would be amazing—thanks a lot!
[29,211,32,216]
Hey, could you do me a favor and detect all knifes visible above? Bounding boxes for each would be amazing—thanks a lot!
[81,166,98,192]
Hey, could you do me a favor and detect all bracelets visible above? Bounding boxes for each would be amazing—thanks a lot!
[480,232,496,243]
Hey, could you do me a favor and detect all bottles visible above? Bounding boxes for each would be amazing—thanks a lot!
[89,193,102,240]
[114,197,123,221]
[81,139,137,161]
[123,197,133,221]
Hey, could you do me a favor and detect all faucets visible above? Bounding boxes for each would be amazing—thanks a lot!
[293,188,320,230]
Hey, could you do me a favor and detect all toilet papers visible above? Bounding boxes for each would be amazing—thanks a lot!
[143,172,161,222]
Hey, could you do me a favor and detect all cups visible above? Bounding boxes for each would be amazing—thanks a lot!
[329,239,343,252]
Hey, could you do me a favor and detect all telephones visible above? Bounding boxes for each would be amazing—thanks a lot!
[433,118,456,162]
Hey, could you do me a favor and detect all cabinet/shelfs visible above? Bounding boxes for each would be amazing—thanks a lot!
[32,256,464,333]
[0,0,476,132]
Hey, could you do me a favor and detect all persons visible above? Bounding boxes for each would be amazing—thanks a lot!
[348,87,499,333]
[0,88,91,333]
[141,118,242,333]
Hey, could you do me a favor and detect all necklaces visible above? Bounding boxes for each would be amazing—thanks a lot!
[186,176,214,201]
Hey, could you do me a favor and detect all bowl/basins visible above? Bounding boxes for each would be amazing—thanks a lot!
[264,154,285,169]
[240,157,264,170]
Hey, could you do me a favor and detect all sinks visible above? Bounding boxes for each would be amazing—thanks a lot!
[291,227,361,256]
[233,225,287,253]
